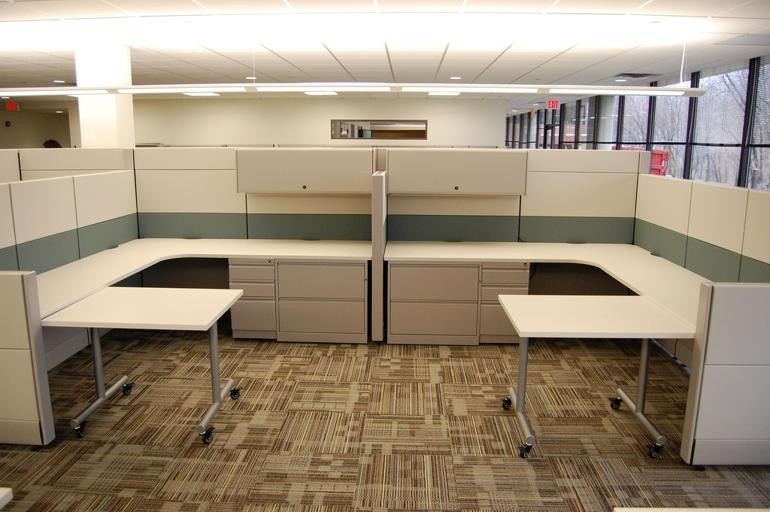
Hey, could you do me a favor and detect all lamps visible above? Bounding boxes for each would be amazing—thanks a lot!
[0,42,709,100]
